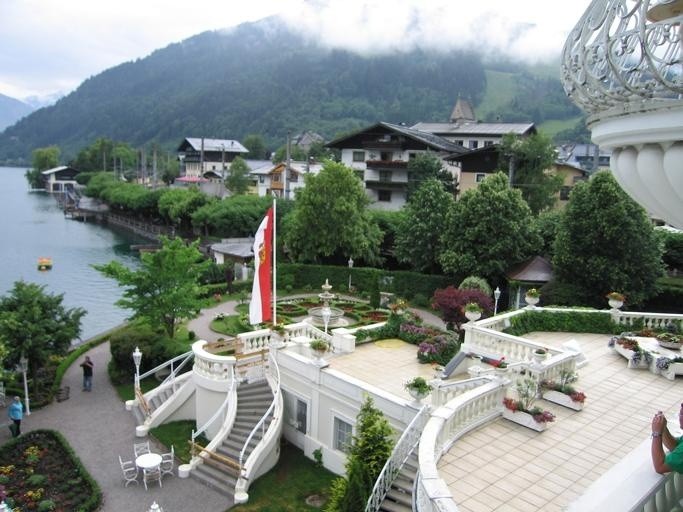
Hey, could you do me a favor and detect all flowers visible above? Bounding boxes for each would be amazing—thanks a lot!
[464,302,484,313]
[605,292,626,302]
[526,287,543,297]
[607,323,683,371]
[480,355,510,369]
[539,368,587,404]
[502,380,556,423]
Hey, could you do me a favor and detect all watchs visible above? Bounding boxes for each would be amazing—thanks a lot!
[652,431,662,437]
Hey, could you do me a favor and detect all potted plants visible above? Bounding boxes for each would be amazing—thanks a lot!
[403,376,434,409]
[532,349,546,366]
[269,324,285,349]
[309,338,335,364]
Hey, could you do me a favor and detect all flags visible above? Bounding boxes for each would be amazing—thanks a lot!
[248,205,272,324]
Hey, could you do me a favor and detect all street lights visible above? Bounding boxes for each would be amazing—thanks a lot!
[0,500,13,512]
[494,286,501,317]
[348,257,354,288]
[132,346,143,406]
[321,301,331,333]
[146,501,164,512]
[19,356,32,417]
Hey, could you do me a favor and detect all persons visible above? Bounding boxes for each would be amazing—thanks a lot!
[8,395,22,434]
[651,403,682,506]
[80,356,93,392]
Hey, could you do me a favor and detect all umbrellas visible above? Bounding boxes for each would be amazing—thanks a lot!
[8,419,18,437]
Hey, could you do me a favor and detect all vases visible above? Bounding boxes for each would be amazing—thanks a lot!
[503,408,547,432]
[608,299,623,312]
[524,295,539,309]
[465,312,482,325]
[542,389,584,411]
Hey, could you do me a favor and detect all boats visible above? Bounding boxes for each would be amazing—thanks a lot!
[37,260,53,270]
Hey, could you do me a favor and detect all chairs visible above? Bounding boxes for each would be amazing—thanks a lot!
[118,440,174,491]
